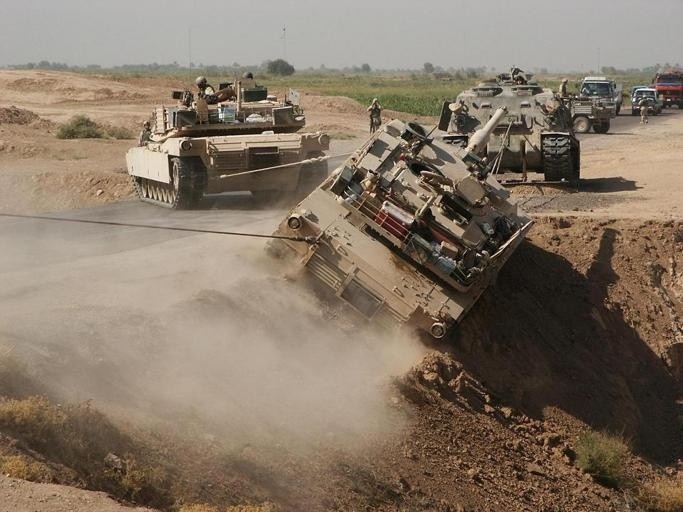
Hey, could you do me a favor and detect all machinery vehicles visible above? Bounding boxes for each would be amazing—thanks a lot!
[263,107,537,344]
[553,91,619,134]
[437,65,581,192]
[125,82,333,210]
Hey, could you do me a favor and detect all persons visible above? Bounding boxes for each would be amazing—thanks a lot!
[366,97,383,132]
[558,79,568,97]
[137,121,150,147]
[193,77,214,105]
[636,97,648,124]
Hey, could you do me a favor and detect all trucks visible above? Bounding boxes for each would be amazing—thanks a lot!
[578,77,624,116]
[630,67,683,116]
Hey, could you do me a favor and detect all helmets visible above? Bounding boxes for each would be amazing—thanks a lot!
[242,71,253,80]
[195,76,207,85]
